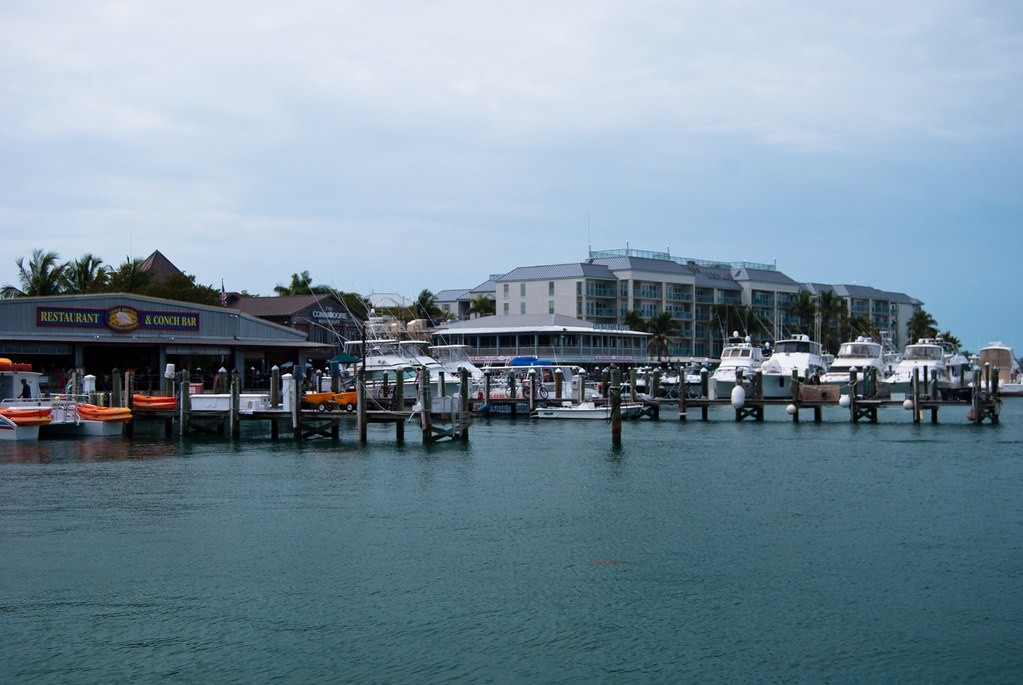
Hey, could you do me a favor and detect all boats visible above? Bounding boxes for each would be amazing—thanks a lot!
[428,343,653,398]
[0,360,134,442]
[529,401,643,420]
[188,390,271,411]
[708,331,762,398]
[659,364,716,395]
[967,341,1023,396]
[620,364,664,393]
[819,336,890,399]
[762,334,829,398]
[316,339,612,399]
[906,332,977,390]
[881,345,952,399]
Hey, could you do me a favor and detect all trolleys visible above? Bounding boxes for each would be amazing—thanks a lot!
[332,390,356,413]
[301,390,335,412]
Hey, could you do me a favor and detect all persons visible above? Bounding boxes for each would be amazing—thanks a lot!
[543,369,549,383]
[414,367,421,396]
[18,378,32,402]
[146,365,153,390]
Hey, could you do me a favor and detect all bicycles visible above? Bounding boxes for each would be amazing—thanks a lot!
[669,379,700,400]
[377,379,397,398]
[524,376,549,399]
